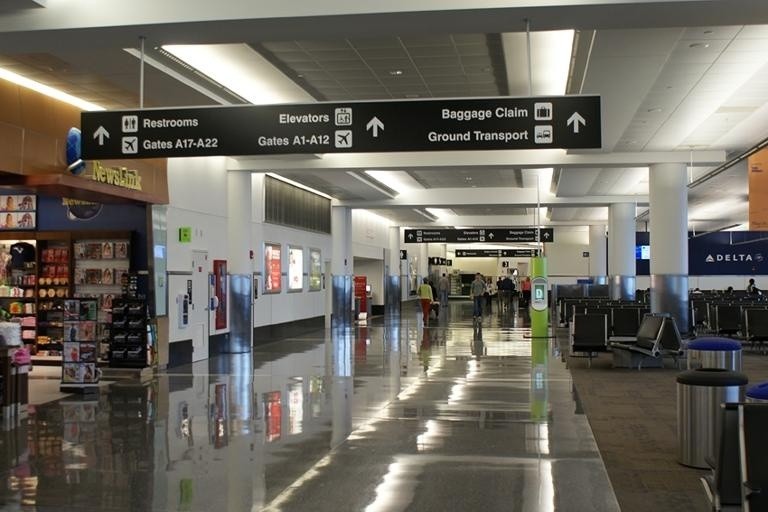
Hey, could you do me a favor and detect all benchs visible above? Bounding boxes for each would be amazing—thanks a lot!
[552,282,768,372]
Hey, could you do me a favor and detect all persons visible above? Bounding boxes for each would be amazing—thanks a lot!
[418,327,433,373]
[469,320,488,369]
[428,279,440,321]
[0,213,16,229]
[119,244,126,255]
[19,197,33,211]
[724,286,736,300]
[65,300,97,382]
[19,214,33,228]
[748,278,756,293]
[0,196,16,211]
[522,277,531,307]
[471,272,516,320]
[646,287,650,303]
[103,242,111,257]
[439,273,449,307]
[694,287,701,294]
[418,278,433,326]
[104,294,113,309]
[103,268,111,284]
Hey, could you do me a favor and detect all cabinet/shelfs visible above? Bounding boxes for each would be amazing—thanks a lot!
[0,231,129,358]
[61,297,97,385]
[110,297,152,367]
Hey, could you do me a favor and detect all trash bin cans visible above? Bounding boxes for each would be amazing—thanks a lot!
[677,368,749,469]
[745,382,767,404]
[687,337,744,370]
